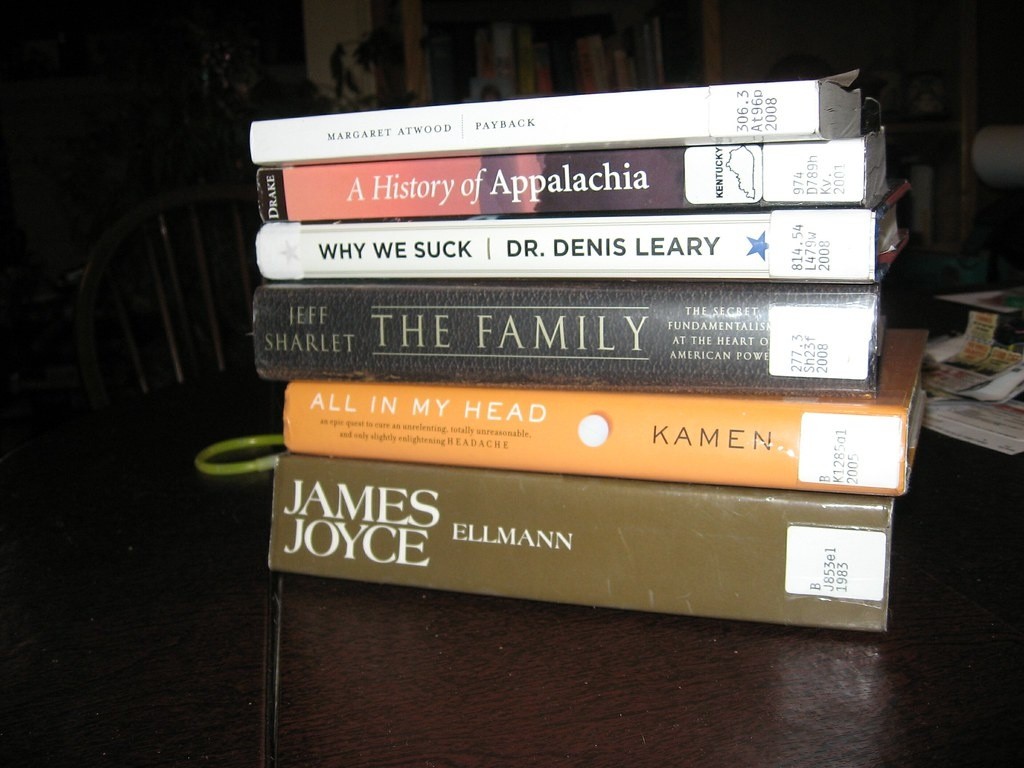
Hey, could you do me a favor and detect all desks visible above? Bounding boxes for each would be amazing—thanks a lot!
[1,368,1024,767]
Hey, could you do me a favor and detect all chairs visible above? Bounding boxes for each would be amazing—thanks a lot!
[68,185,260,408]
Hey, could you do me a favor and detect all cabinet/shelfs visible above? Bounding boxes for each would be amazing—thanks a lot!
[701,1,981,254]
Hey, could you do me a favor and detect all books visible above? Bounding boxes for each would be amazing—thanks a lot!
[249,70,931,632]
